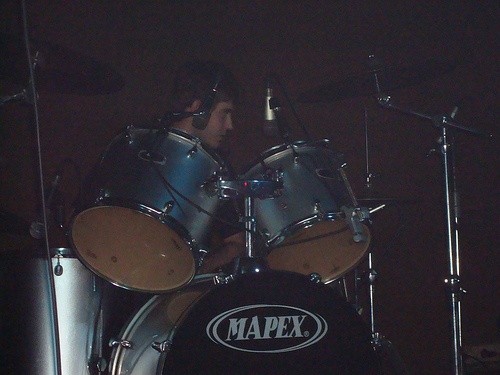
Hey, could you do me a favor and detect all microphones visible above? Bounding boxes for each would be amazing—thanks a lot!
[263,78,278,136]
[191,77,228,130]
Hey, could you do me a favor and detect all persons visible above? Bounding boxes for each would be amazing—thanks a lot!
[62,62,253,282]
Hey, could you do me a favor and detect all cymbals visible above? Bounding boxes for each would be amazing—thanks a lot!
[0,34,126,98]
[353,195,430,206]
[297,57,457,102]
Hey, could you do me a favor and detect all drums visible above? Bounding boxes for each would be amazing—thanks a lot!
[110,267,383,375]
[0,246,113,375]
[69,122,233,294]
[236,138,372,286]
